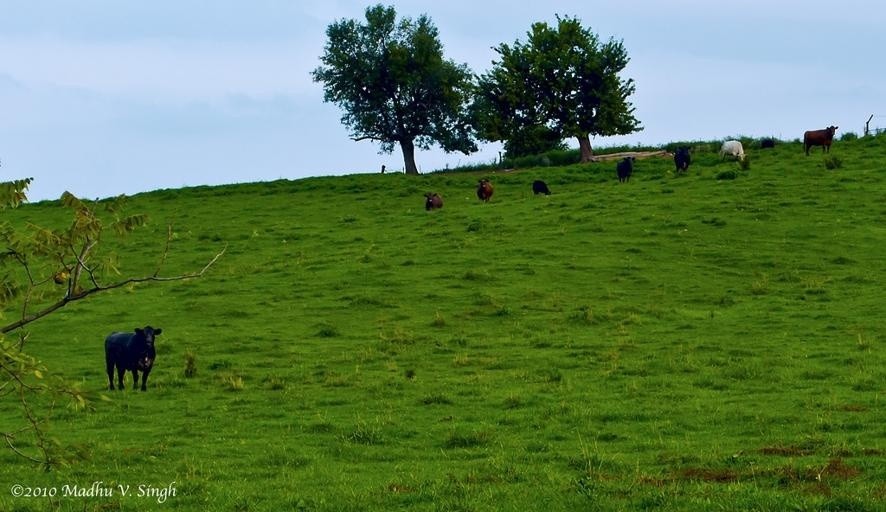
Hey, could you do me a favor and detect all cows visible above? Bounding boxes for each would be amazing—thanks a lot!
[718,140,749,162]
[533,180,551,195]
[674,145,691,173]
[424,191,443,211]
[105,326,162,391]
[477,179,493,203]
[617,157,635,184]
[804,126,838,156]
